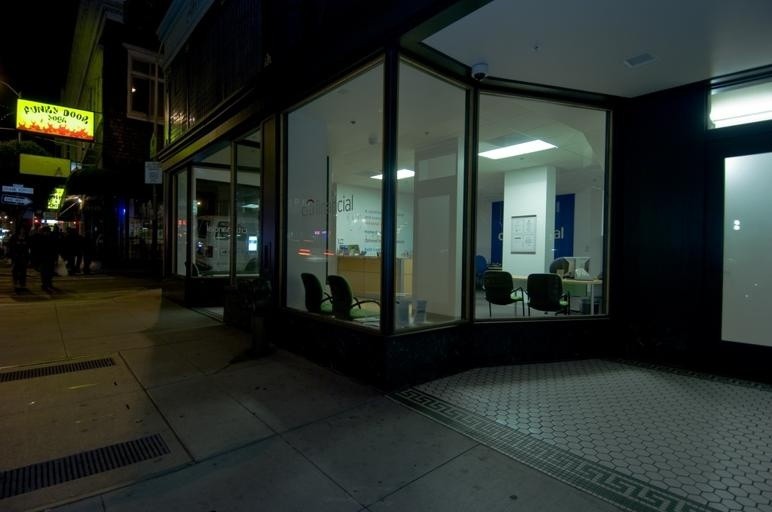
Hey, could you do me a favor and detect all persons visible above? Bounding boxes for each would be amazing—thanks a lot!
[2,223,96,290]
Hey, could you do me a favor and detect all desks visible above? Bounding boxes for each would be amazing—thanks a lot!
[336,253,413,303]
[505,271,604,316]
[354,309,461,332]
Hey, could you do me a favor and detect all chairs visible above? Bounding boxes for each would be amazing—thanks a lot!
[480,268,527,317]
[243,258,258,271]
[183,261,200,277]
[301,272,336,316]
[524,274,570,316]
[325,274,382,320]
[475,254,487,289]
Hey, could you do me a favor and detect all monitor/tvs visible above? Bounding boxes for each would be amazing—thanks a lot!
[248,234,258,251]
